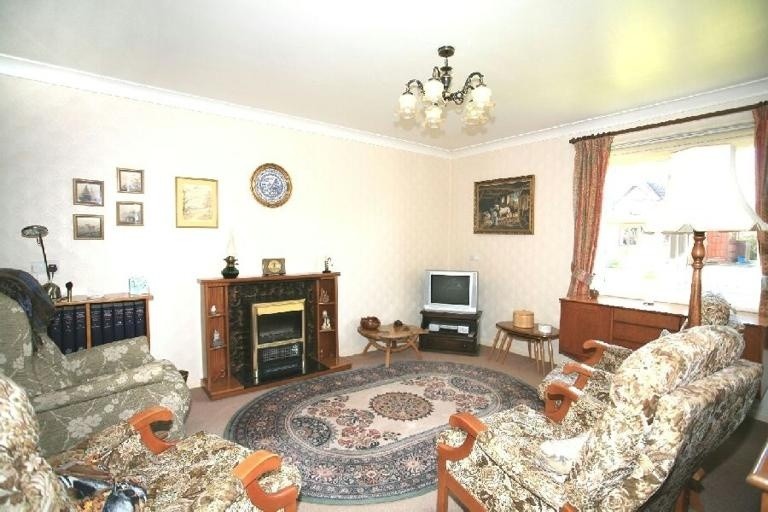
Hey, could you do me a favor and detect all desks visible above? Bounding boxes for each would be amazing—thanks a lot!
[357,323,428,369]
[490,319,559,377]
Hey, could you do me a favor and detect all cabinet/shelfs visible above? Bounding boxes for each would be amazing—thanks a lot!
[559,294,768,402]
[201,272,353,401]
[418,310,482,356]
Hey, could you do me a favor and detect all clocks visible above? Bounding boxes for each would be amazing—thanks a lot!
[262,259,286,277]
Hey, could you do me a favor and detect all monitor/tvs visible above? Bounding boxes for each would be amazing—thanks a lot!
[423,270,478,315]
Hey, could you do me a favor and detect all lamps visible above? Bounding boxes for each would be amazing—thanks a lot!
[644,148,768,329]
[393,46,496,128]
[21,225,62,300]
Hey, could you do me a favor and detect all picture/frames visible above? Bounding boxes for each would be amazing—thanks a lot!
[175,177,219,228]
[250,163,292,208]
[116,201,143,226]
[73,214,104,240]
[117,167,145,194]
[73,178,104,207]
[619,221,646,248]
[473,175,535,234]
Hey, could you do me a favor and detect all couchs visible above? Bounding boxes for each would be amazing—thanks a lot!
[0,268,301,512]
[438,326,763,511]
[564,295,745,411]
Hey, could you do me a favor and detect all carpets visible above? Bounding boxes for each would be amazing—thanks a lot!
[222,361,544,505]
